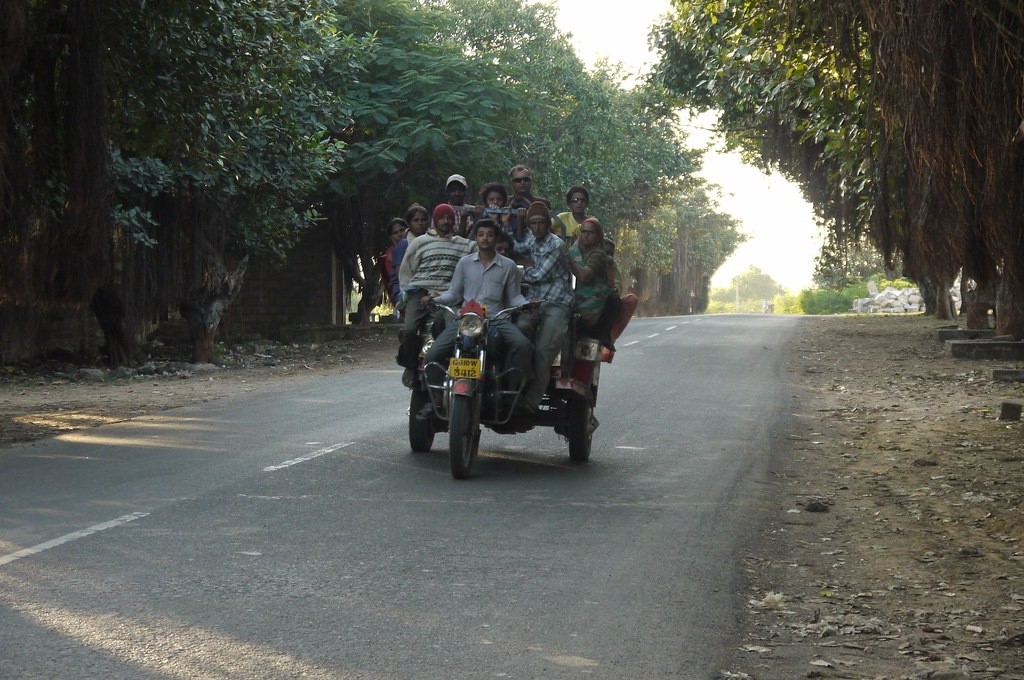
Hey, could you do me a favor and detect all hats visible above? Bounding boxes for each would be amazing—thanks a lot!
[433,204,456,226]
[527,201,551,227]
[446,174,467,189]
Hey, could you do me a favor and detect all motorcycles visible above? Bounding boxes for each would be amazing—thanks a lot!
[408,300,600,480]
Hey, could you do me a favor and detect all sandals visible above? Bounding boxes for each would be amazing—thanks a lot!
[416,403,440,421]
[402,369,418,387]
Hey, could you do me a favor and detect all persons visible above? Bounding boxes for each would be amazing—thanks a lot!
[414,220,536,422]
[383,164,638,418]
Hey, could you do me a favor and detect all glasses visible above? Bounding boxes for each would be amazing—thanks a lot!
[512,177,530,182]
[571,197,587,203]
